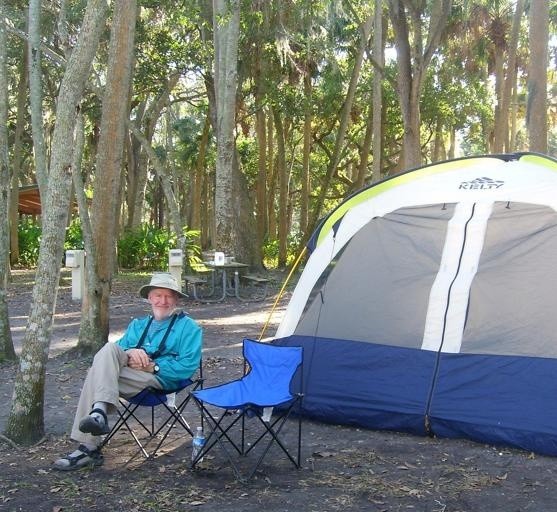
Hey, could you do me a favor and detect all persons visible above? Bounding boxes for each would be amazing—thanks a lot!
[50,274,205,474]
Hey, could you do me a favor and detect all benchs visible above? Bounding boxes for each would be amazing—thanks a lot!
[184,276,207,284]
[241,275,269,282]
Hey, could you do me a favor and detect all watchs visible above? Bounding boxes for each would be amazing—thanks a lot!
[153,363,159,375]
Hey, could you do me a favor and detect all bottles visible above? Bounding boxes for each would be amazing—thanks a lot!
[192,427,206,463]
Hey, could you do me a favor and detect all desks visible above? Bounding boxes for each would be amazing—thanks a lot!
[185,262,267,304]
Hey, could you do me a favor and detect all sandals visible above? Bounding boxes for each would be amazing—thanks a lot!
[77,407,112,437]
[51,443,105,472]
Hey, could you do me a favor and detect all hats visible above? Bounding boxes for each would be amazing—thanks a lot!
[137,271,190,300]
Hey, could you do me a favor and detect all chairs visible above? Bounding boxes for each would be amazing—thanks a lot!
[100,356,206,461]
[185,338,304,488]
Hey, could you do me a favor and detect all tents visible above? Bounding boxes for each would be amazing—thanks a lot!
[240,149,557,457]
